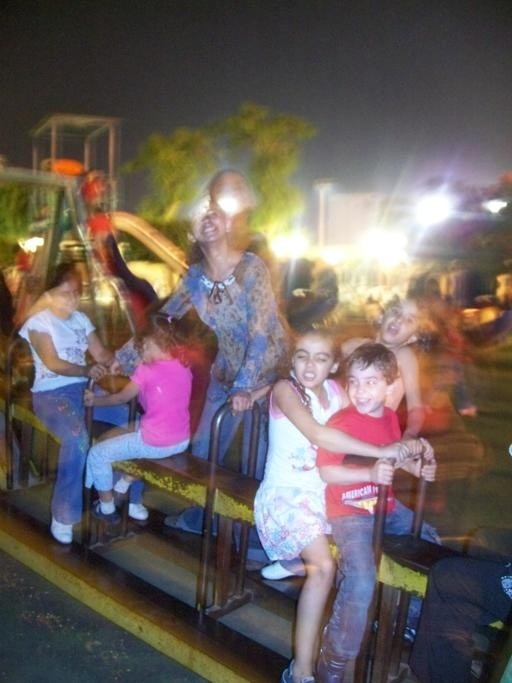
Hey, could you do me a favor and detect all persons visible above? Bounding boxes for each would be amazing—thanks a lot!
[252,297,512,683]
[0,199,299,545]
